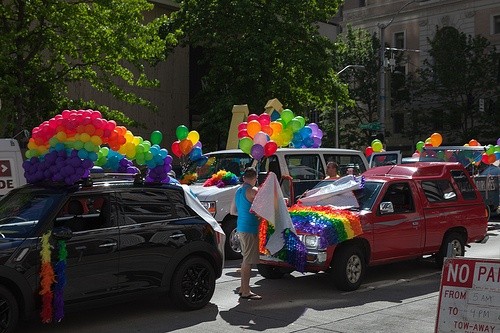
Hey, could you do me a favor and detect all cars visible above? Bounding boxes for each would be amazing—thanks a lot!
[463,148,500,215]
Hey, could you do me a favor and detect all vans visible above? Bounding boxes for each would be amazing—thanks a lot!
[386,146,499,172]
[180,148,371,260]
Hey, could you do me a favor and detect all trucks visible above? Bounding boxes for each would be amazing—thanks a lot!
[0,138,139,199]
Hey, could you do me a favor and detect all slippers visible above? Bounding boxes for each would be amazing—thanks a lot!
[238,290,263,299]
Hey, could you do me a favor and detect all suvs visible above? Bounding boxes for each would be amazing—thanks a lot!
[251,162,491,292]
[0,178,223,333]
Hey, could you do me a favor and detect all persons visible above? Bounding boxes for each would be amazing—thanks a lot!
[324,161,339,180]
[230,167,263,300]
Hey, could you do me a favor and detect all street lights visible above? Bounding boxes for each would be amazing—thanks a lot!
[333,65,366,162]
[386,47,422,134]
[376,0,431,153]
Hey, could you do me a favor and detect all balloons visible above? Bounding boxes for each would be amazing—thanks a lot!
[365,139,386,162]
[238,109,323,160]
[412,132,500,167]
[22,109,216,185]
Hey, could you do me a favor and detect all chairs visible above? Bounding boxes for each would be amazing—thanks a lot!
[387,189,410,213]
[68,200,85,230]
